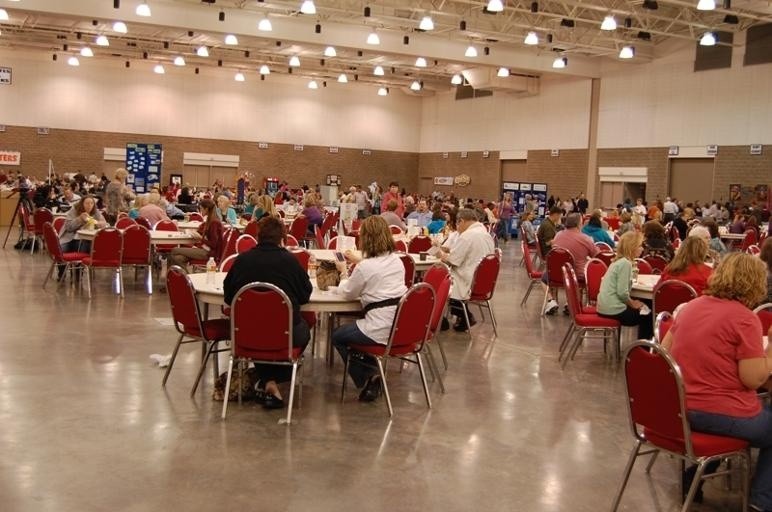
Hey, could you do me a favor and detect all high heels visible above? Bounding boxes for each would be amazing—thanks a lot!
[359,375,385,400]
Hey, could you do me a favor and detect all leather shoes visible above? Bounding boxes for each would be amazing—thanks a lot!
[255,388,284,408]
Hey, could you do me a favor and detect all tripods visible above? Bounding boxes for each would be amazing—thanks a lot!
[2,187,33,249]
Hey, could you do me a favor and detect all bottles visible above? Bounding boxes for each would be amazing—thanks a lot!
[307,253,317,288]
[205,256,217,286]
[631,262,639,283]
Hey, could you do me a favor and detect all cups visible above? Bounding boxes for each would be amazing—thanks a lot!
[184,216,189,223]
[52,207,57,214]
[419,251,427,261]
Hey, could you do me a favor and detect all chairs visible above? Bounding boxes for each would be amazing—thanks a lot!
[560,265,622,373]
[161,265,234,398]
[608,335,754,512]
[221,281,307,423]
[4,178,770,302]
[459,254,502,340]
[421,261,455,369]
[643,310,744,494]
[655,311,672,346]
[652,278,696,339]
[751,303,770,339]
[339,282,436,422]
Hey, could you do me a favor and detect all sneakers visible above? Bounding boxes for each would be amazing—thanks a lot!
[454,313,477,330]
[545,300,570,315]
[682,471,704,503]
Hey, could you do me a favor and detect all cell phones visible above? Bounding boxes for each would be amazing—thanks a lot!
[333,251,345,262]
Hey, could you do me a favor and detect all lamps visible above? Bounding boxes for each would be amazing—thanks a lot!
[53,0,726,99]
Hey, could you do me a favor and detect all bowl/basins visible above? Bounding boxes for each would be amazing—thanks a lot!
[60,206,72,212]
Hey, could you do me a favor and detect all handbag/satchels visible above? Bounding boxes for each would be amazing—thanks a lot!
[214,367,256,401]
[316,259,350,290]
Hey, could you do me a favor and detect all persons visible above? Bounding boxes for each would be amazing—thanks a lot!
[335,214,409,399]
[223,215,314,407]
[1,168,324,291]
[660,251,772,512]
[341,180,519,330]
[522,192,772,349]
